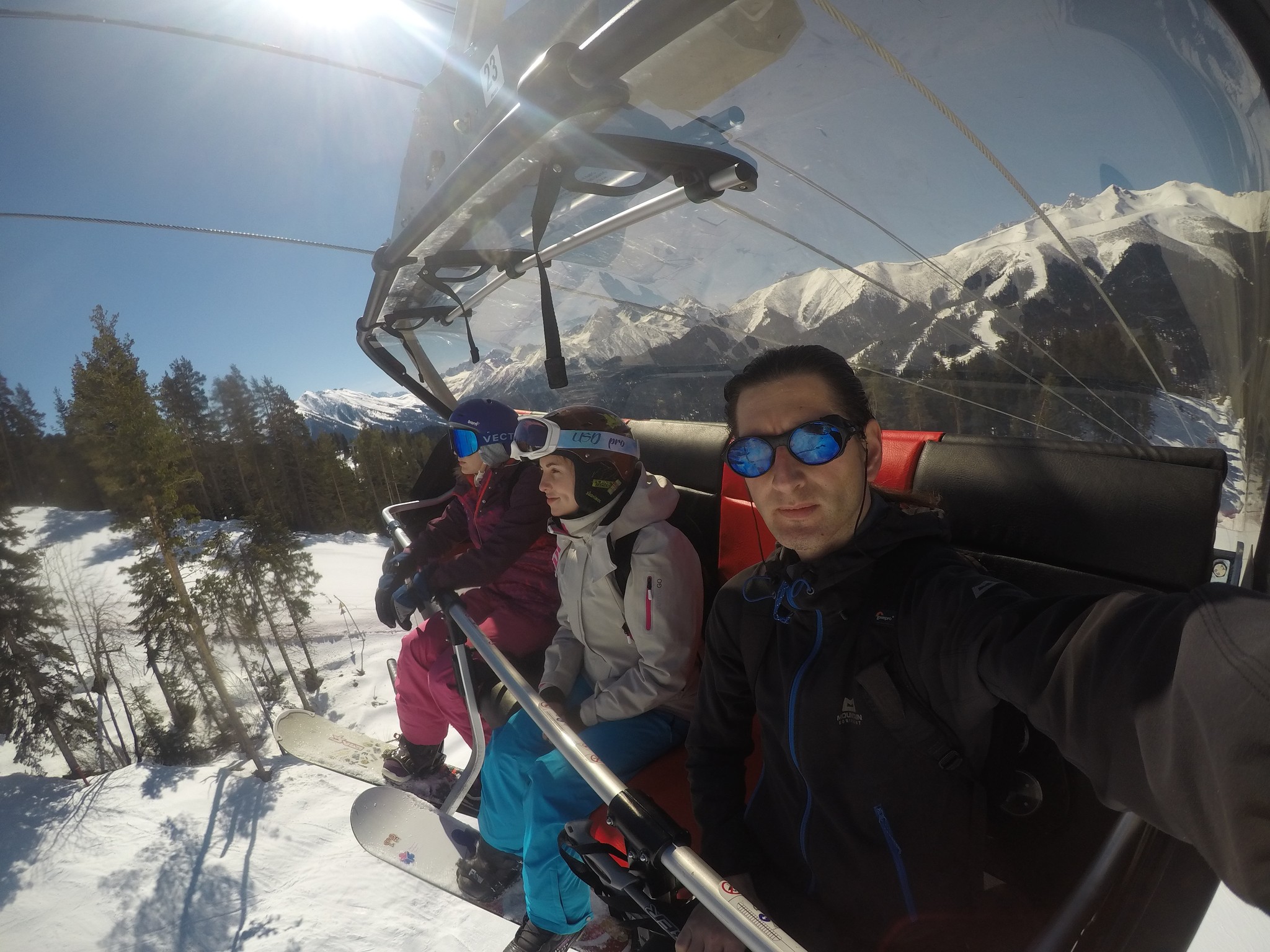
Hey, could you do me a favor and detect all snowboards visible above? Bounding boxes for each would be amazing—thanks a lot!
[350,786,632,952]
[272,708,483,819]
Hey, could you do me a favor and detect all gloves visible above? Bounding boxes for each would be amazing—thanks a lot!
[375,552,418,629]
[390,573,432,631]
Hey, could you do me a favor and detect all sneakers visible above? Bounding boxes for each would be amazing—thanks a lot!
[455,773,483,811]
[502,911,586,952]
[457,839,524,903]
[383,735,447,782]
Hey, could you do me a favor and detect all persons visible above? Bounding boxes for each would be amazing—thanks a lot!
[674,345,1270,952]
[457,405,704,952]
[375,398,562,813]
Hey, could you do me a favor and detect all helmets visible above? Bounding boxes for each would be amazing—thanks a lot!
[542,404,638,511]
[448,398,518,458]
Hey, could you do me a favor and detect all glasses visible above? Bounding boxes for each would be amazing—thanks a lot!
[718,414,865,479]
[509,415,560,462]
[448,429,481,457]
